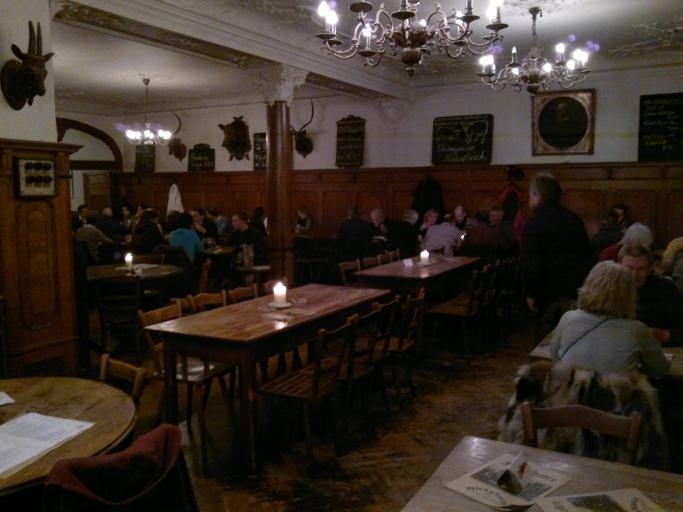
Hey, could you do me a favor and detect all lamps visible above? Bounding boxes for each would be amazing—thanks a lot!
[124,79,172,146]
[312,1,509,83]
[473,6,592,99]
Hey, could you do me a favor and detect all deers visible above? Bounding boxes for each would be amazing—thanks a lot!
[167,112,187,162]
[289,99,315,159]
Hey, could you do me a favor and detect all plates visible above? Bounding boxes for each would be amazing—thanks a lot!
[417,262,431,265]
[269,302,291,308]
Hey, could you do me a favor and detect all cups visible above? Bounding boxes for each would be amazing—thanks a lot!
[125,256,132,266]
[207,236,215,249]
[272,286,286,305]
[420,252,429,263]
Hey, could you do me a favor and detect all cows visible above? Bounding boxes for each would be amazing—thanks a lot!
[219,118,249,157]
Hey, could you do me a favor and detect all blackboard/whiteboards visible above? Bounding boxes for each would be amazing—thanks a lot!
[135,145,154,173]
[188,149,215,174]
[253,132,266,172]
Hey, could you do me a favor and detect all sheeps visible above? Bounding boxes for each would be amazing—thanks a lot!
[10,20,55,106]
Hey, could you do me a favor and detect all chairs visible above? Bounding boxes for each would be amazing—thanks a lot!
[494,352,672,472]
[38,423,200,512]
[82,230,510,459]
[520,400,644,467]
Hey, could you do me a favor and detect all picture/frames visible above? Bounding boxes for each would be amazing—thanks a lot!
[133,144,155,172]
[187,143,215,171]
[252,132,266,170]
[530,88,595,155]
